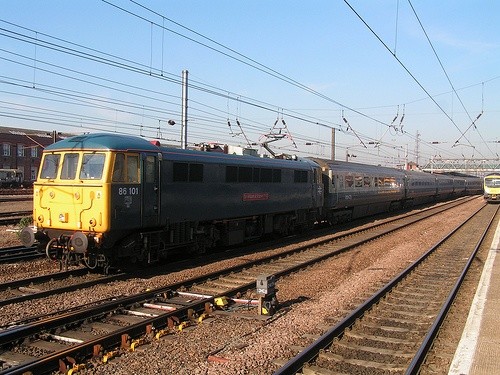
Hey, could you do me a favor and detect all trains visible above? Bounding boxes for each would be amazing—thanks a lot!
[17,131,485,275]
[483,171,500,206]
